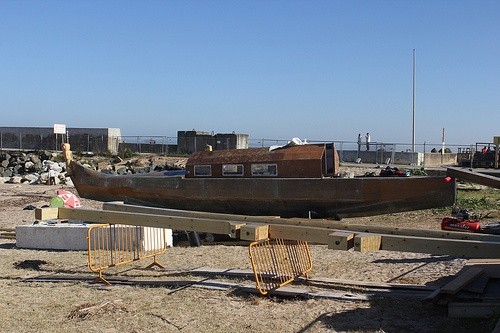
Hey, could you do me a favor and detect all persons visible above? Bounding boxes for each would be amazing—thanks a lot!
[429,143,500,156]
[379,166,394,177]
[392,166,405,175]
[365,132,371,151]
[356,133,362,152]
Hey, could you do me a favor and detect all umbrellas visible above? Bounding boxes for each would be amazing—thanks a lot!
[57,189,84,209]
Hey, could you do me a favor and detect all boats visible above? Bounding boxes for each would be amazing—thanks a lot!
[70,142,458,224]
